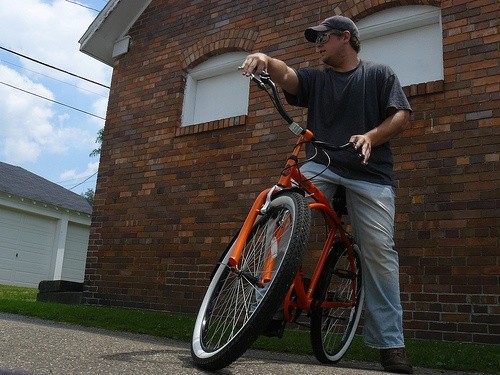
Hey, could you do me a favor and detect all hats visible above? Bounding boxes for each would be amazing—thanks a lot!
[304,16,360,44]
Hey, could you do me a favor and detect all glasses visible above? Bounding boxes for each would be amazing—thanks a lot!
[316,33,338,43]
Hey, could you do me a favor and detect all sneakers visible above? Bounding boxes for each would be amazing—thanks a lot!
[380,348,413,374]
[233,319,282,336]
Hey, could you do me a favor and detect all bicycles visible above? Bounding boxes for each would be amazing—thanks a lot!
[190,66,368,374]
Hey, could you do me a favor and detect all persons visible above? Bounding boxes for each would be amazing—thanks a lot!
[241,15,414,374]
